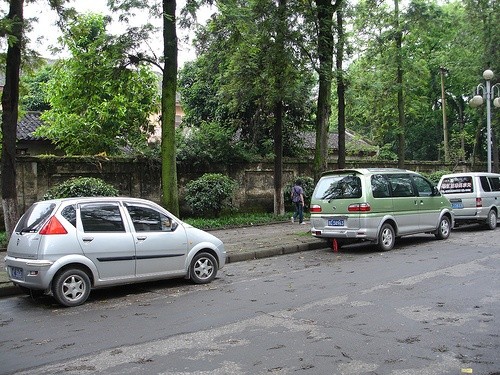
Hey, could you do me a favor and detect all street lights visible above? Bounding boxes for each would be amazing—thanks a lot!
[469,70,500,172]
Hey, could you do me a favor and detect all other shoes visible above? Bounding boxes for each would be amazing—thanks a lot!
[291,217,295,224]
[298,221,306,225]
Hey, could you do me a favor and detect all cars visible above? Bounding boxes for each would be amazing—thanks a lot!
[4,197,226,307]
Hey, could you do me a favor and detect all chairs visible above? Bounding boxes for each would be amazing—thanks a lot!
[331,185,410,198]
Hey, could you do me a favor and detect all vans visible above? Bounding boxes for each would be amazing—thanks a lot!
[310,168,456,251]
[438,172,500,230]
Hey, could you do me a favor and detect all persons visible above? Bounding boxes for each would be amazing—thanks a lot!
[290,179,305,225]
